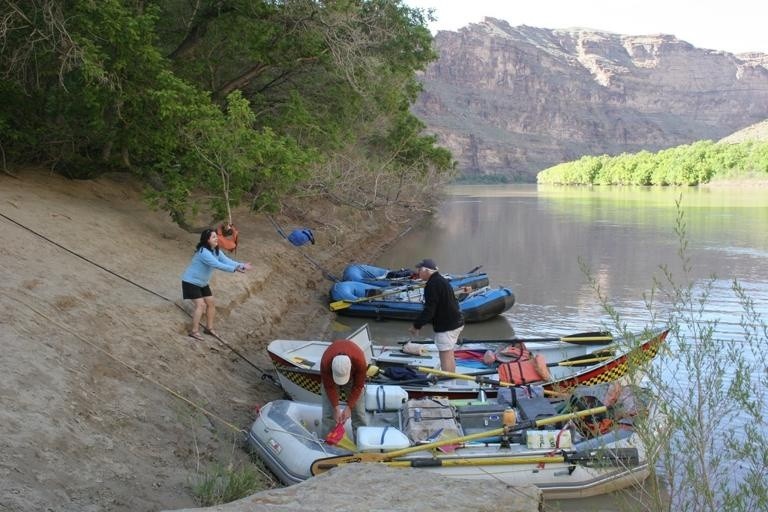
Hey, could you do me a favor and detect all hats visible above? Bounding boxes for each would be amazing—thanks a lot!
[415,258,437,270]
[331,355,352,386]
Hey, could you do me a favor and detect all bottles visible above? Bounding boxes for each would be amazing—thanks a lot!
[503,407,515,427]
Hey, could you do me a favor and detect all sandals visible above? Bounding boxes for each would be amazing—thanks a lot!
[203,327,218,339]
[188,330,205,341]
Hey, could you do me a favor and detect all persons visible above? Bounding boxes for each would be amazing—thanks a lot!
[408,258,464,372]
[319,339,366,446]
[181,229,258,341]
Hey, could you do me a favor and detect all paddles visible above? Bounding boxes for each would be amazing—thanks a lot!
[318,448,638,469]
[329,284,425,312]
[310,404,613,478]
[397,332,612,345]
[388,352,615,387]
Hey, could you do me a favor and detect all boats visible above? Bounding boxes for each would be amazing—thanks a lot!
[329,264,514,324]
